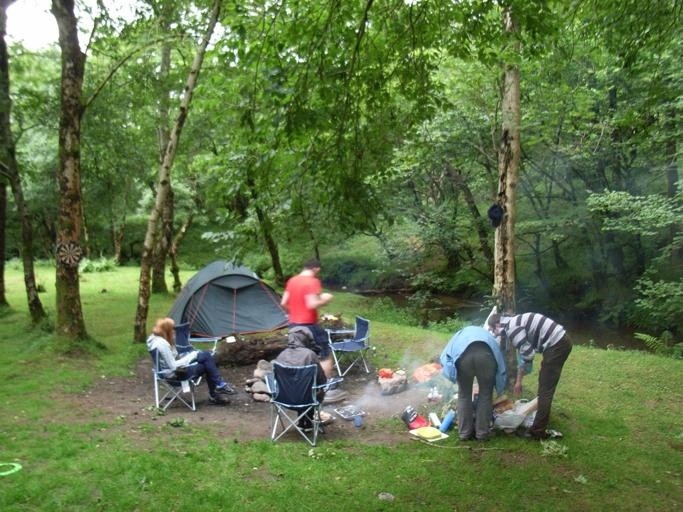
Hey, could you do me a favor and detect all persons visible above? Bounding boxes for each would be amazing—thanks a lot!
[144,315,238,408]
[267,326,325,434]
[438,325,506,440]
[488,309,573,441]
[276,258,345,403]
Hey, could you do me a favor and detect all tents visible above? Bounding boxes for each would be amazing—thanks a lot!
[164,257,287,341]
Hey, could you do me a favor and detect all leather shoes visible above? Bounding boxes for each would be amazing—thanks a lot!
[515,431,535,438]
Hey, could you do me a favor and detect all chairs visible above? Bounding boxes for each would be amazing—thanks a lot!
[323,314,374,381]
[143,346,201,419]
[172,321,218,388]
[262,360,346,448]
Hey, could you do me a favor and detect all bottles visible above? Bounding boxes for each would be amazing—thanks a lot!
[438,408,456,434]
[428,412,442,428]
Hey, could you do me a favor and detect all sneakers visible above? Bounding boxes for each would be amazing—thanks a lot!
[214,382,238,396]
[207,395,231,405]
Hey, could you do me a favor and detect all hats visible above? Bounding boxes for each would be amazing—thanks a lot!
[487,205,503,228]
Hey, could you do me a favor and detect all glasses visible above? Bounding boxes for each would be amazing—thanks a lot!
[491,328,495,336]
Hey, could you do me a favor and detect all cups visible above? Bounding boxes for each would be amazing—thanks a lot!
[353,416,362,427]
